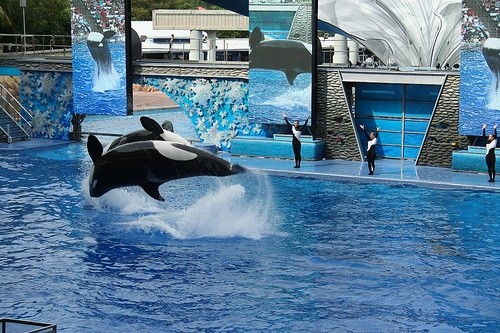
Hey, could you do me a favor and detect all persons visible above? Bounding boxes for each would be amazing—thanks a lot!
[482,0,500,30]
[282,113,310,168]
[70,1,125,37]
[483,123,497,182]
[462,2,490,43]
[360,125,380,175]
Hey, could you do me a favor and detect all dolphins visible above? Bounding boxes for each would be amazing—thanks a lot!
[249,26,312,86]
[87,30,117,80]
[479,27,500,95]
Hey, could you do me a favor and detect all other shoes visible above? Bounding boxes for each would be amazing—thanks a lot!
[293,166,300,168]
[487,179,495,182]
[368,172,374,175]
